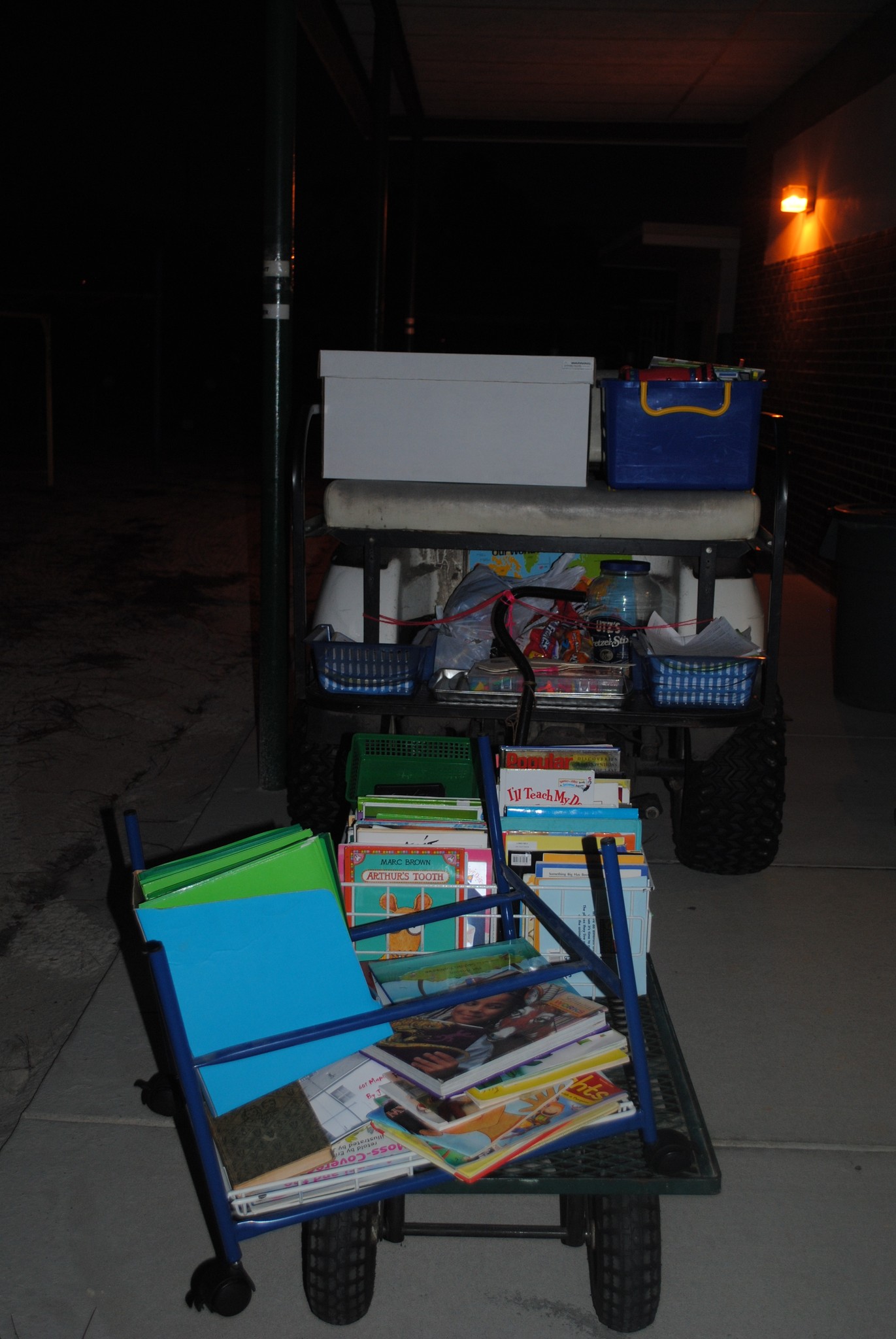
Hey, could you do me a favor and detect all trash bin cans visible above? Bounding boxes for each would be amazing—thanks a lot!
[829,503,894,712]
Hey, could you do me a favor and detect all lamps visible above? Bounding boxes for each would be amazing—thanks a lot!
[780,184,813,213]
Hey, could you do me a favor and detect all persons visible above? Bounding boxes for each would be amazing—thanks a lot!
[375,983,530,1080]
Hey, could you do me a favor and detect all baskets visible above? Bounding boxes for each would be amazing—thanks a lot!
[344,733,477,805]
[304,639,422,698]
[633,639,758,710]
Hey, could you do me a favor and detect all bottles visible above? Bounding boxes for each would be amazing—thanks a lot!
[586,559,664,682]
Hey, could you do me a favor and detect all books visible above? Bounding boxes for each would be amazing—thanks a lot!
[130,730,651,1227]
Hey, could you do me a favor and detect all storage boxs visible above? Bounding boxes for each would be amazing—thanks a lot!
[601,378,767,492]
[321,350,596,487]
